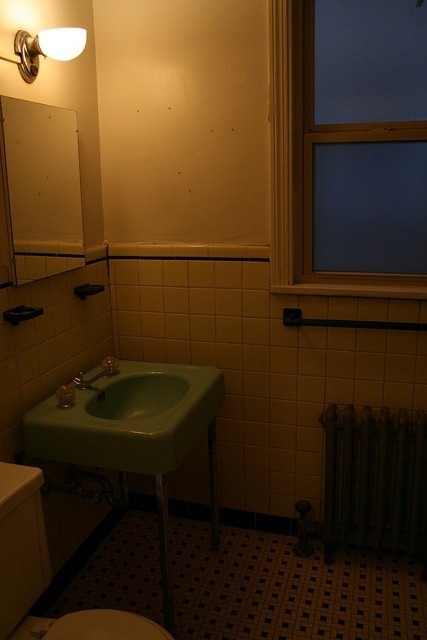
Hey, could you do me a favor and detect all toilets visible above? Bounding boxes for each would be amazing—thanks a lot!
[1,462,176,640]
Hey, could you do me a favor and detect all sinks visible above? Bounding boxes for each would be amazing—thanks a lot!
[85,372,191,422]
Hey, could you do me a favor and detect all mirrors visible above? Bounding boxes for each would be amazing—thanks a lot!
[0,94,86,286]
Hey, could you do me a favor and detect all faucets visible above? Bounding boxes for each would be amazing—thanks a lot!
[72,367,115,389]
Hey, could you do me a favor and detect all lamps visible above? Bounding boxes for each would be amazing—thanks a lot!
[14,25,87,83]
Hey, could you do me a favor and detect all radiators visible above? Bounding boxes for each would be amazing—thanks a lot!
[294,405,427,565]
